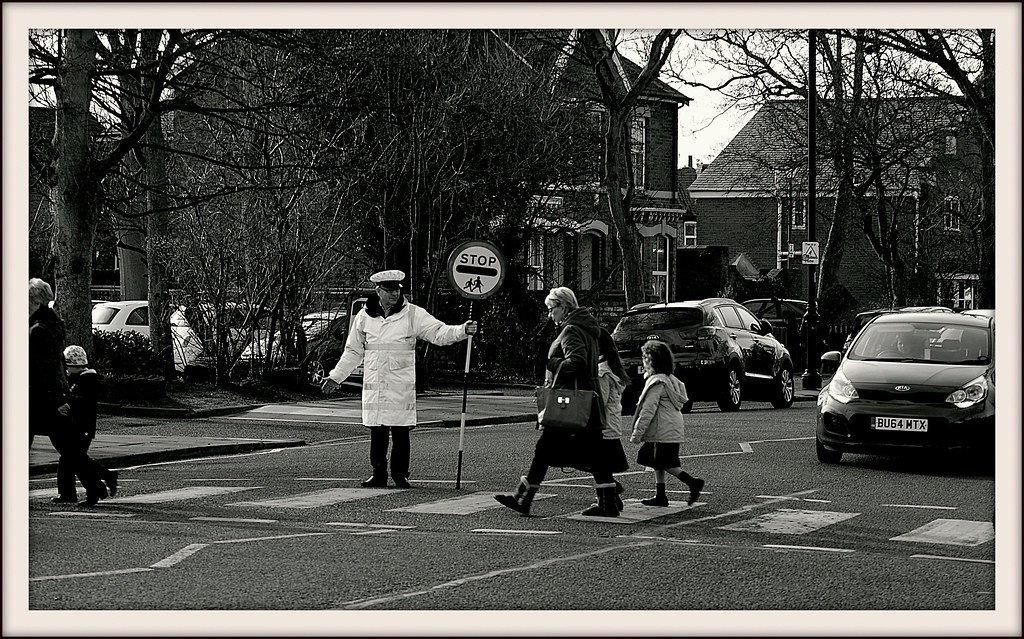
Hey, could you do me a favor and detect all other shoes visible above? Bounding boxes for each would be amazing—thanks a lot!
[361,473,388,486]
[106,470,118,496]
[641,495,669,506]
[51,491,78,503]
[688,478,705,506]
[391,475,412,488]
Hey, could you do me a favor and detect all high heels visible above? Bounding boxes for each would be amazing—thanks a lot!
[79,481,109,507]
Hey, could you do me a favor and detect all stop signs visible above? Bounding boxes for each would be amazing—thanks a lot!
[446,239,507,299]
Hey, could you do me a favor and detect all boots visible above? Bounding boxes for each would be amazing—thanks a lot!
[494,476,540,514]
[582,482,620,517]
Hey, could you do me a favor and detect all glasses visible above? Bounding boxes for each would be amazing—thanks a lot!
[546,303,561,313]
[379,285,400,293]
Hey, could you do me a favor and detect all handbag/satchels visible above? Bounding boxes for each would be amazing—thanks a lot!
[535,361,593,427]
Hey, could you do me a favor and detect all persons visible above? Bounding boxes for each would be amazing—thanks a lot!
[492,286,628,517]
[320,269,477,487]
[28,277,107,508]
[50,345,119,502]
[629,340,704,505]
[891,332,918,356]
[799,301,820,375]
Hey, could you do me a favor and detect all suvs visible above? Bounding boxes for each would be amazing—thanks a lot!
[609,297,795,416]
[739,298,810,333]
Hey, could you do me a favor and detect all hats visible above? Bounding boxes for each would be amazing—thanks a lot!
[63,345,88,364]
[370,270,406,289]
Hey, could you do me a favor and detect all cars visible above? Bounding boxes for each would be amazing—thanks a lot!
[816,306,996,474]
[47,300,347,395]
